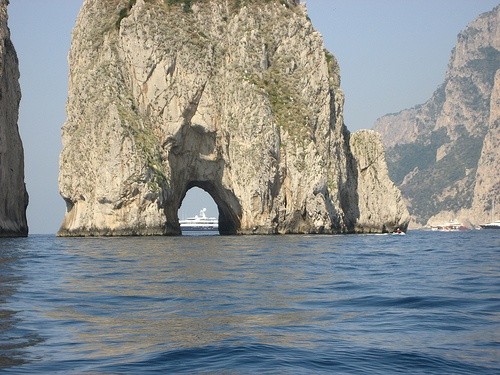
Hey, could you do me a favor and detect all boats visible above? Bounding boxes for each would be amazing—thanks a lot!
[431,223,464,231]
[480,221,500,229]
[393,230,406,236]
[179,208,218,231]
